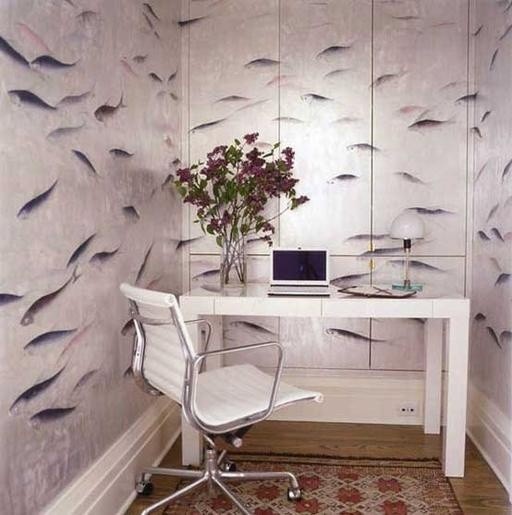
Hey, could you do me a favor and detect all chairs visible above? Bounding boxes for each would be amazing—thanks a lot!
[118,281,325,515]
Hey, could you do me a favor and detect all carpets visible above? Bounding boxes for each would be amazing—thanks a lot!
[160,449,464,515]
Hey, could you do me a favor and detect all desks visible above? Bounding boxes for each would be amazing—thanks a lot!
[178,284,470,479]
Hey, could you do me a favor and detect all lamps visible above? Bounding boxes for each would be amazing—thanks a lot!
[388,212,425,292]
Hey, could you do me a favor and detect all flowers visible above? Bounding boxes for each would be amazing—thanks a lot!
[169,131,311,284]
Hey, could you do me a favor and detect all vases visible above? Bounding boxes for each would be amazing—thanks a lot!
[219,234,248,288]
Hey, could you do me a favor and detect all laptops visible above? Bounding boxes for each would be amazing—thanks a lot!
[267,248,331,295]
